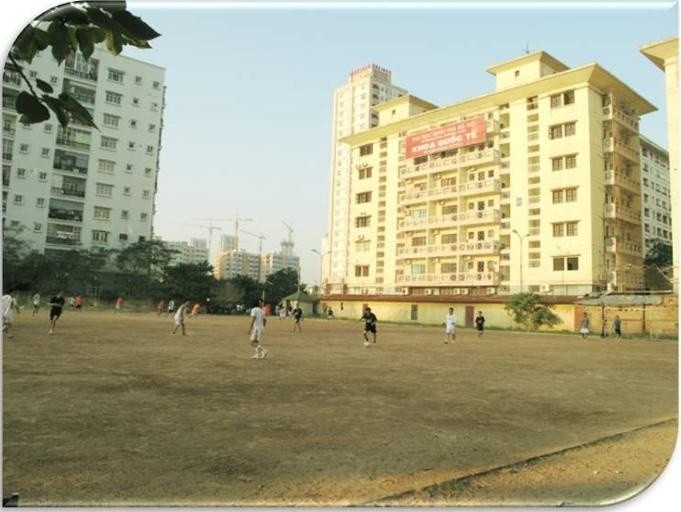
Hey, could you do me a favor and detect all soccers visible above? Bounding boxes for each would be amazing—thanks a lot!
[363,341,371,348]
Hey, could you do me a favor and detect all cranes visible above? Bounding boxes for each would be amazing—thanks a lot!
[198,214,252,252]
[238,229,267,254]
[282,220,294,244]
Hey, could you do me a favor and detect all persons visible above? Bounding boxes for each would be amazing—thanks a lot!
[156,298,201,336]
[358,307,377,347]
[2,290,20,338]
[30,290,82,335]
[474,311,485,338]
[249,298,269,359]
[443,308,457,344]
[114,295,123,316]
[578,312,591,341]
[611,315,622,342]
[327,307,335,319]
[262,302,305,334]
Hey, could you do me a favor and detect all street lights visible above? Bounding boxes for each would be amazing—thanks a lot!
[511,230,531,293]
[311,248,333,290]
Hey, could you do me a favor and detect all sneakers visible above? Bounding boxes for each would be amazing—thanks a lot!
[262,349,268,359]
[253,354,260,358]
